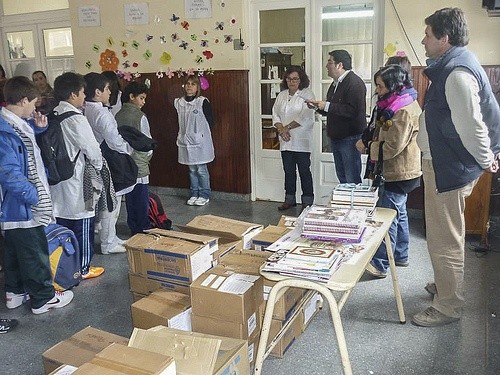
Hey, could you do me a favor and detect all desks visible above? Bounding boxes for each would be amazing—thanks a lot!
[464,169,493,245]
[253,204,406,375]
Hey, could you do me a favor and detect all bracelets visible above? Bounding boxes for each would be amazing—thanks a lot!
[286,125,292,130]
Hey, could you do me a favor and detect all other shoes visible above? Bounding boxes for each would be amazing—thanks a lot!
[81,266,104,281]
[195,196,209,206]
[0,318,19,335]
[426,282,437,295]
[186,196,198,205]
[366,263,387,278]
[101,239,126,254]
[412,306,455,326]
[395,259,409,267]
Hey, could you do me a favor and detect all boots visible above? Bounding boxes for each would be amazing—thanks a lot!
[300,195,315,214]
[278,194,297,210]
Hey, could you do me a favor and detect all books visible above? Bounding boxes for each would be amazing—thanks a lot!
[262,183,380,283]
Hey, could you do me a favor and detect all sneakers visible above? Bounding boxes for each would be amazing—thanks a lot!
[31,290,74,316]
[5,291,30,308]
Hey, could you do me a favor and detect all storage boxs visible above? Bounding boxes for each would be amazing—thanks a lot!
[41,214,323,375]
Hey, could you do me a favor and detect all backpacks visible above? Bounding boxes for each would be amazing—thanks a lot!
[43,224,83,293]
[35,109,81,185]
[148,192,172,230]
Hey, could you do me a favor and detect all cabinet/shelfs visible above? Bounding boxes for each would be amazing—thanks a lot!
[260,53,294,115]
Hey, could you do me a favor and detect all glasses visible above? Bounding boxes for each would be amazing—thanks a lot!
[285,77,299,83]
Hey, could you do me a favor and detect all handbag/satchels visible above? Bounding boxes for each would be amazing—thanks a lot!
[372,175,384,206]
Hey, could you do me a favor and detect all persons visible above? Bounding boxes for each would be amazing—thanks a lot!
[0,318,19,333]
[357,57,422,278]
[173,76,215,206]
[304,50,367,184]
[32,71,154,279]
[0,76,73,315]
[412,9,500,327]
[273,64,315,213]
